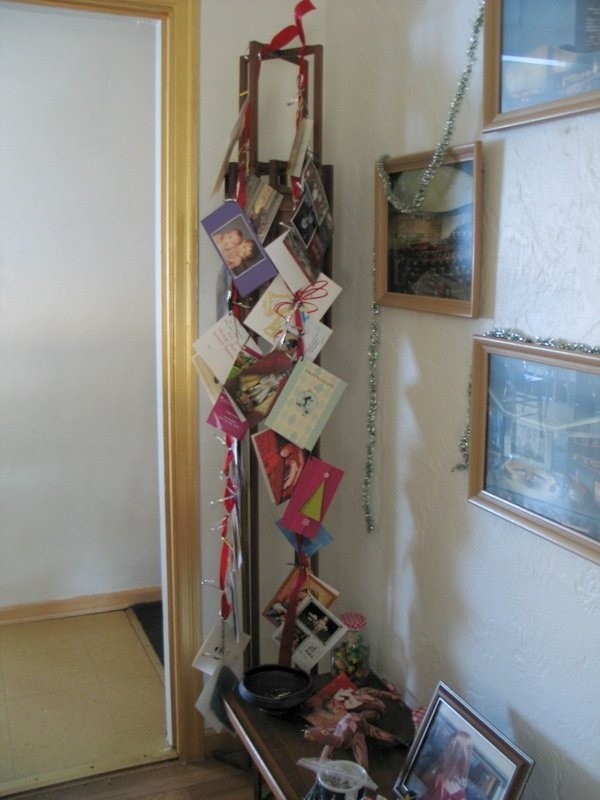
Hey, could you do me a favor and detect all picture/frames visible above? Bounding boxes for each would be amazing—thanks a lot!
[393,680,534,800]
[467,334,600,566]
[373,142,480,318]
[481,0,600,132]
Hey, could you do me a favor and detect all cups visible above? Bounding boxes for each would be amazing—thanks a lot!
[316,760,368,800]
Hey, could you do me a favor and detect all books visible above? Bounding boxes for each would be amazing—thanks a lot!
[190,159,366,731]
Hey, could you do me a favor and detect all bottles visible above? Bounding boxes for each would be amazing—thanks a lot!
[331,613,371,686]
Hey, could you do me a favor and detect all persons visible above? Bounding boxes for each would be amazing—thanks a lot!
[417,732,474,800]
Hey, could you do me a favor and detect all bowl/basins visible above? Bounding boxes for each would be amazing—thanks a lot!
[238,665,315,714]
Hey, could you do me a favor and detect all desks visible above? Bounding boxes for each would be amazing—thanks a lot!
[217,667,417,800]
